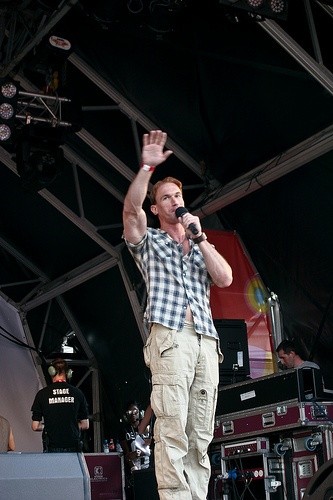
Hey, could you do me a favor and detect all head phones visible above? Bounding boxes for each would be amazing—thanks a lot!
[48,360,73,379]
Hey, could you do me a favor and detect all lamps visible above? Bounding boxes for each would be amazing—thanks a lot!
[220,0,288,21]
[39,35,74,60]
[0,79,19,142]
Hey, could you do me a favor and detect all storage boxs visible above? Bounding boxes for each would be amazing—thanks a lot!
[85,365,333,500]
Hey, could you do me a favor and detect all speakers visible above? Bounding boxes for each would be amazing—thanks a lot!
[213,319,250,376]
[301,457,333,500]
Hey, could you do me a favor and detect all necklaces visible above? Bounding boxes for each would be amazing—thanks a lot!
[180,238,186,246]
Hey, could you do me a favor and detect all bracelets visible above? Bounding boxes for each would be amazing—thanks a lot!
[142,164,154,171]
[192,232,207,244]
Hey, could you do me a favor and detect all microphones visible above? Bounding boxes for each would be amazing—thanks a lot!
[174,207,199,236]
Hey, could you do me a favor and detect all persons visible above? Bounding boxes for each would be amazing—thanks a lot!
[0,416,14,452]
[123,403,155,469]
[30,359,89,453]
[276,340,319,369]
[121,130,232,500]
